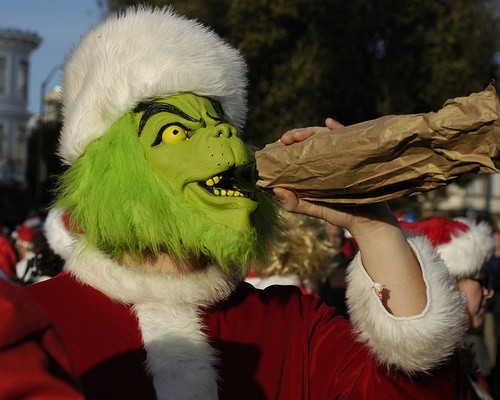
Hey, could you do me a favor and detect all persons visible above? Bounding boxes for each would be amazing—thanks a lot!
[0,80,480,400]
[0,204,499,400]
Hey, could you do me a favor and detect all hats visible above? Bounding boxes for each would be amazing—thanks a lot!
[12,215,44,249]
[52,3,250,161]
[44,196,95,260]
[346,211,496,277]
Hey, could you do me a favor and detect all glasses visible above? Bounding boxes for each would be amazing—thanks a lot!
[457,270,490,288]
